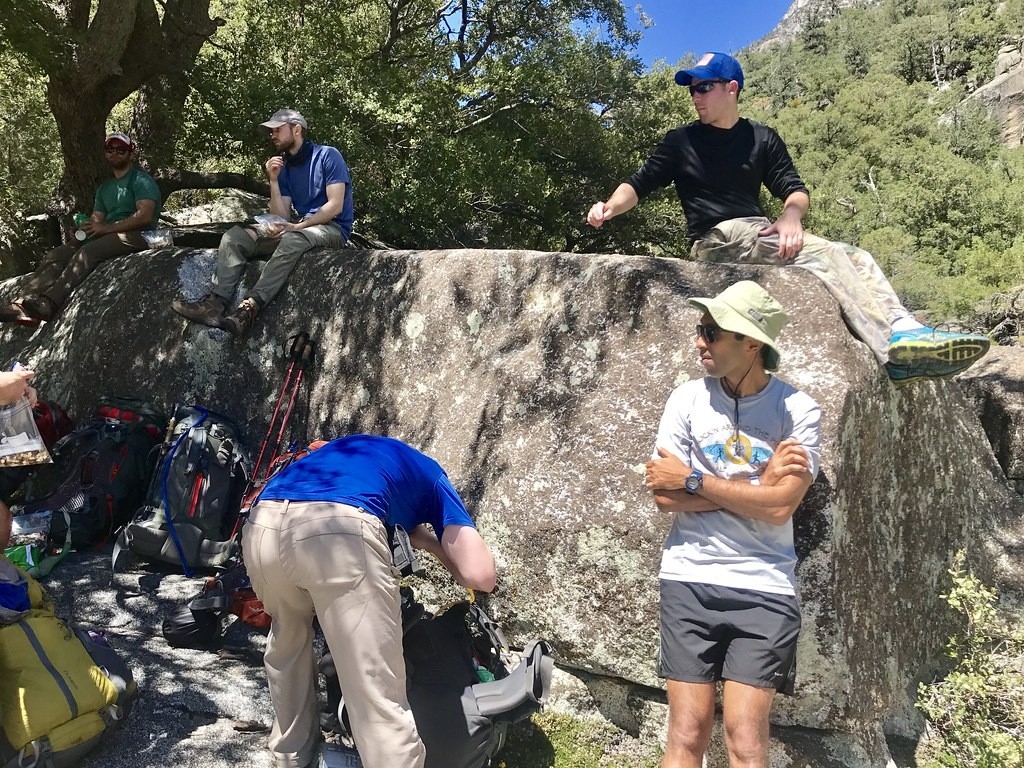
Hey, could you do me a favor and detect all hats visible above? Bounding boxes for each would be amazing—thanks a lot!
[259,109,308,135]
[675,52,744,89]
[105,132,132,151]
[685,280,786,372]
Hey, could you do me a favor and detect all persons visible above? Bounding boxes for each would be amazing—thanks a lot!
[170,110,354,336]
[645,280,824,768]
[587,51,991,386]
[0,372,37,554]
[0,130,160,325]
[219,435,498,768]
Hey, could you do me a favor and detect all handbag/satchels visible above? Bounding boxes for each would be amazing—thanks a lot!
[29,400,72,447]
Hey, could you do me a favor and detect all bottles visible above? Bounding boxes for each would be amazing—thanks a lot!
[75,213,96,244]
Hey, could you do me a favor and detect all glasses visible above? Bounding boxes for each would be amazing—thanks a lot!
[105,146,126,154]
[690,80,728,97]
[697,325,735,343]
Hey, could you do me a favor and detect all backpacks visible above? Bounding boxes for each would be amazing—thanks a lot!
[118,406,250,568]
[19,403,169,556]
[203,440,330,633]
[401,599,555,768]
[0,554,126,768]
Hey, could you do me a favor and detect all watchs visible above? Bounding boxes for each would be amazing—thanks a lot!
[684,469,704,495]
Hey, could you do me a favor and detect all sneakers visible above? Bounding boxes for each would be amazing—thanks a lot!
[888,323,991,366]
[172,293,228,326]
[221,300,258,335]
[886,361,973,385]
[0,302,38,327]
[22,295,56,322]
